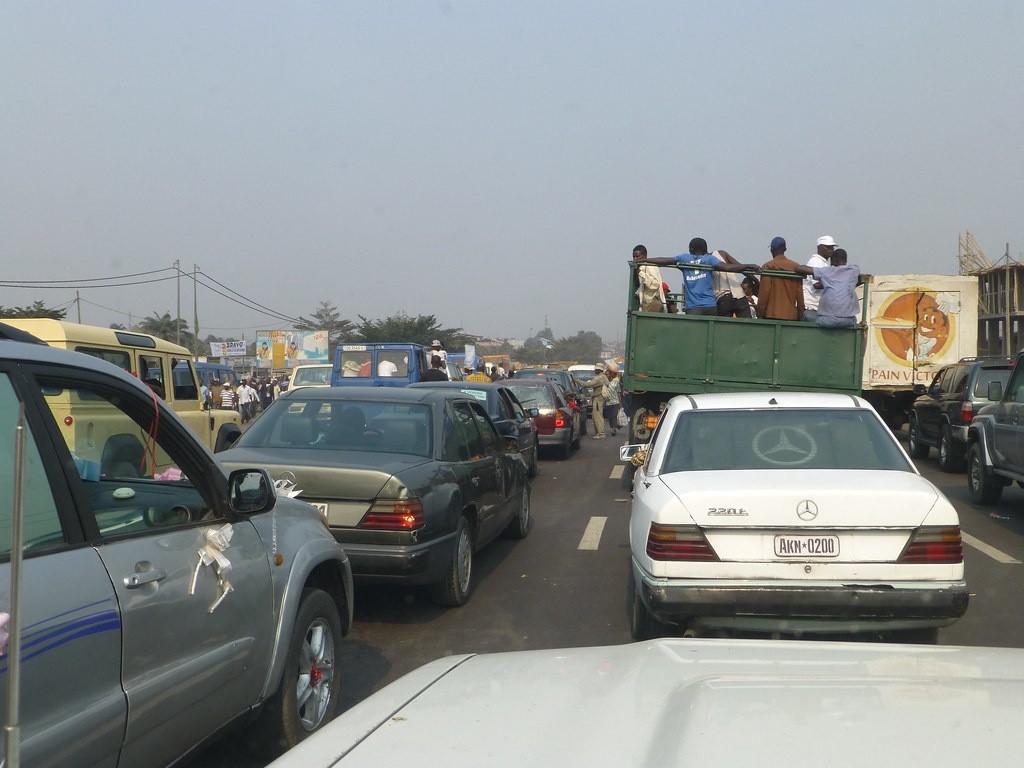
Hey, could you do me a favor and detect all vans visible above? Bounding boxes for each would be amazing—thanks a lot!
[568,365,596,414]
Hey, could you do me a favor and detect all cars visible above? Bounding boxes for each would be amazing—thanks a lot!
[492,379,582,460]
[208,385,529,605]
[618,392,970,643]
[268,635,1024,768]
[376,381,539,478]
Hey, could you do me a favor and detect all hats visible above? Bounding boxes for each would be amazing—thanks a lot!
[431,340,443,347]
[595,363,604,371]
[464,363,475,370]
[223,383,230,386]
[817,236,837,246]
[768,237,785,247]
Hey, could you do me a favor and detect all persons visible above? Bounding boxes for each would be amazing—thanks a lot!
[801,236,839,322]
[573,362,631,440]
[341,338,514,383]
[756,237,804,321]
[633,234,758,320]
[795,249,861,330]
[196,373,281,424]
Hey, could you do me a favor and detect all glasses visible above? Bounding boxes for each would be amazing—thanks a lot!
[664,289,670,293]
[214,381,218,382]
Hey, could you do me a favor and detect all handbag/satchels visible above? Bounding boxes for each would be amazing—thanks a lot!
[601,386,611,402]
[617,407,628,426]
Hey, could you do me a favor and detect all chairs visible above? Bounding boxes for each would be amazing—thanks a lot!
[527,392,549,408]
[149,378,163,399]
[289,407,426,452]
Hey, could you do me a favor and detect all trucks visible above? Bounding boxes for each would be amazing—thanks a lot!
[853,275,978,426]
[623,258,871,452]
[332,342,429,418]
[446,354,486,380]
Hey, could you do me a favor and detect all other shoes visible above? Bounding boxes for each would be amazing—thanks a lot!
[241,419,244,423]
[611,430,616,435]
[592,433,605,438]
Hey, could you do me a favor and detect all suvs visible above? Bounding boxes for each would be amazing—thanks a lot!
[0,317,242,483]
[966,350,1024,502]
[909,356,1020,472]
[0,322,354,768]
[512,369,589,433]
[288,363,334,421]
